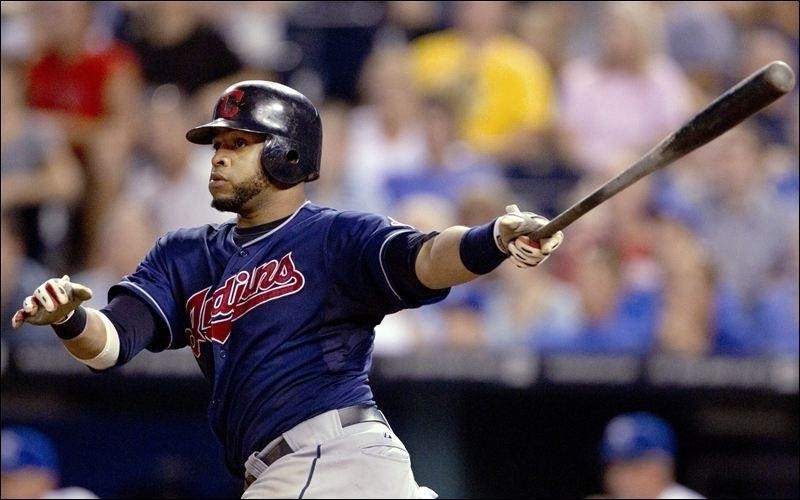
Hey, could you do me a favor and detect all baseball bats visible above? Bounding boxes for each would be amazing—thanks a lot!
[509,60,795,257]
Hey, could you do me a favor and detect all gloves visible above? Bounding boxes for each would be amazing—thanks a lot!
[12,275,91,329]
[494,204,564,271]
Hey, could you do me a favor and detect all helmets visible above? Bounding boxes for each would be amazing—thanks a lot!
[602,413,676,467]
[185,80,323,186]
[0,427,62,484]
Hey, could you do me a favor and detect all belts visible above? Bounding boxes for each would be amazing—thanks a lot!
[242,405,391,491]
